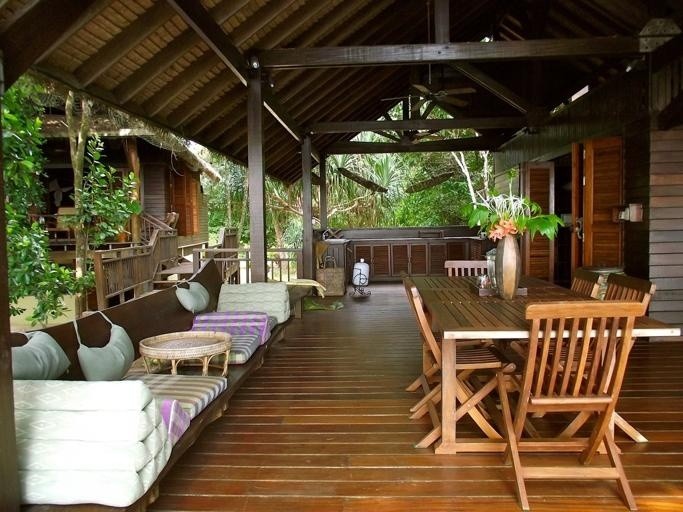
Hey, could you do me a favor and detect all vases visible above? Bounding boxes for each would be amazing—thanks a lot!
[495,233,523,299]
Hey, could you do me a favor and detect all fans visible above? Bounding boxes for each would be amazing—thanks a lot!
[380,1,478,112]
[372,90,444,147]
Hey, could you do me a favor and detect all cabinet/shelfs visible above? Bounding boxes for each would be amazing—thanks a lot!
[322,238,353,285]
[428,242,468,277]
[390,243,428,276]
[353,243,391,277]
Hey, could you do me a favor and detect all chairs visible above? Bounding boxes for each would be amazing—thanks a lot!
[506,268,605,391]
[496,299,647,512]
[144,211,181,240]
[400,270,502,411]
[46,206,77,239]
[445,259,489,277]
[406,279,514,450]
[510,272,658,454]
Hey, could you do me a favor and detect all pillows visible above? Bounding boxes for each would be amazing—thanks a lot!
[10,330,70,379]
[174,280,211,315]
[76,325,138,379]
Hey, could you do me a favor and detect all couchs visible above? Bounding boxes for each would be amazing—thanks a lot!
[11,258,295,512]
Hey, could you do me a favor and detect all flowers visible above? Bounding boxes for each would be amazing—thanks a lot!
[459,167,565,241]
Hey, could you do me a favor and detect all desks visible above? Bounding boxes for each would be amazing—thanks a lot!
[410,273,681,457]
[138,330,231,378]
